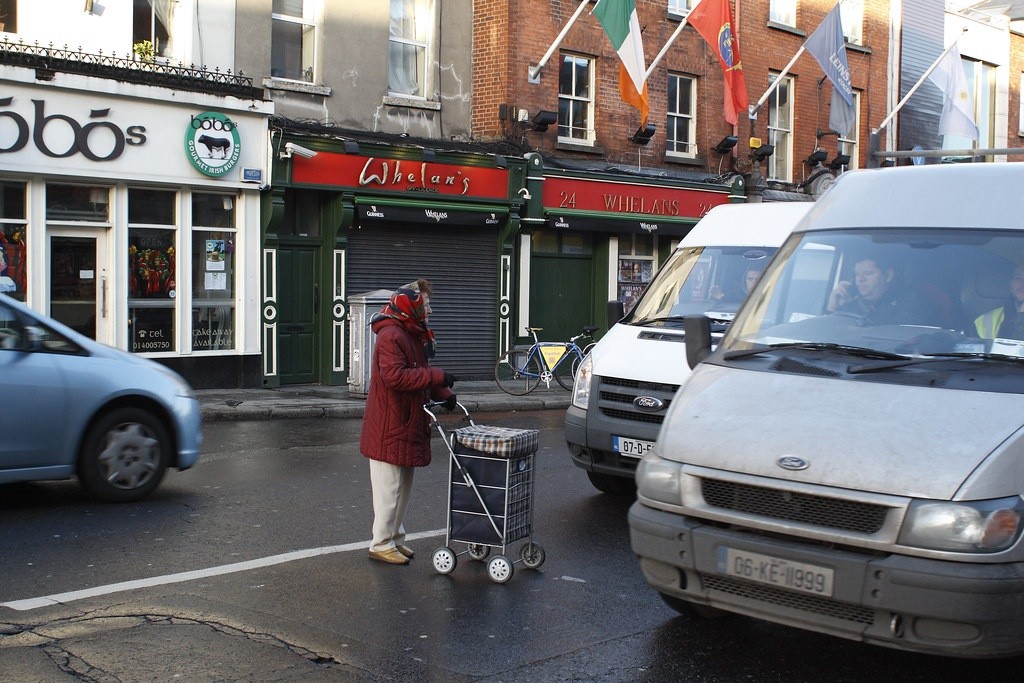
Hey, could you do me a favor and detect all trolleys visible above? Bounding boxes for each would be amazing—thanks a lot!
[423,398,546,583]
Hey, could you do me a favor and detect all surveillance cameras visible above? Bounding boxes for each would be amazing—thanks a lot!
[285,143,318,158]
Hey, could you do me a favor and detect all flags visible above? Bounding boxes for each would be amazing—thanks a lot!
[592,0,650,132]
[803,1,856,136]
[928,45,978,141]
[686,0,749,126]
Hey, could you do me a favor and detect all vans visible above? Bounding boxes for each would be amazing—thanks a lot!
[564,146,1023,661]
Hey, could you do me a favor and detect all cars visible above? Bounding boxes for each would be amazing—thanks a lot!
[0,293,202,504]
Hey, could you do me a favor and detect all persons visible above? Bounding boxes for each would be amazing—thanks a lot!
[966,262,1024,341]
[821,254,925,325]
[359,277,456,562]
[709,266,765,302]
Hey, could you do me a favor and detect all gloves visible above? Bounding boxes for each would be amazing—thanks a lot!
[444,371,457,389]
[443,394,456,411]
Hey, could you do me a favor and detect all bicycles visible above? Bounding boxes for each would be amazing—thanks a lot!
[494,326,599,396]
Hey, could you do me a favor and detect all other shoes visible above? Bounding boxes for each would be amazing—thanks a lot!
[368,546,409,565]
[396,545,414,557]
